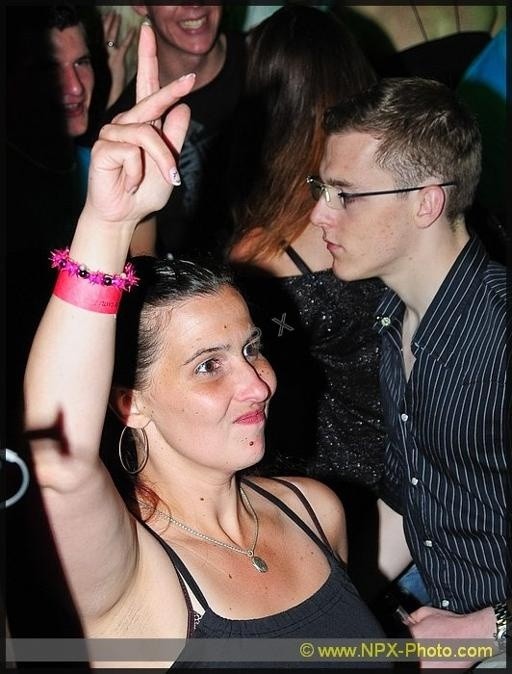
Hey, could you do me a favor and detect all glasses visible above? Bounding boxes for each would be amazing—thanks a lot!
[306,173,457,210]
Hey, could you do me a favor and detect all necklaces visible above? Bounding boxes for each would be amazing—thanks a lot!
[128,486,268,573]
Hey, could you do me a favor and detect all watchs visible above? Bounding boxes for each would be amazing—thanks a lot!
[492,600,512,655]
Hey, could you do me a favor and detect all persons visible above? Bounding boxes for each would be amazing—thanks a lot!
[311,77,512,669]
[94,2,246,267]
[220,2,401,501]
[352,4,506,93]
[24,18,395,673]
[92,6,152,112]
[3,2,156,259]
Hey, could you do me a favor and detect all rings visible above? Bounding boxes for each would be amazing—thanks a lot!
[107,42,116,47]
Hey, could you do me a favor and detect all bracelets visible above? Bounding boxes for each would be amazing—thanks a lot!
[51,250,139,290]
[54,269,123,315]
